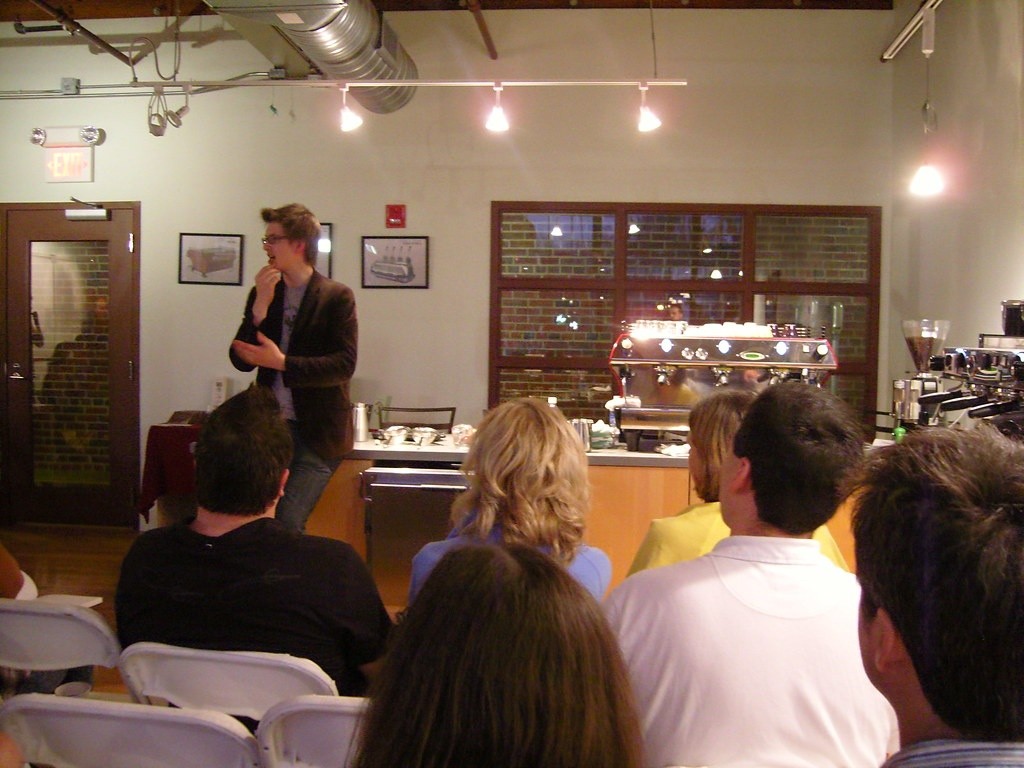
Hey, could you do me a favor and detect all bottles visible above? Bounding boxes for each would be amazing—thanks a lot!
[572,419,590,451]
[609,396,621,427]
[548,397,557,408]
[351,402,368,442]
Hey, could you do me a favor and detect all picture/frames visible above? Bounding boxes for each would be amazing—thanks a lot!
[314,222,334,280]
[176,233,245,288]
[362,234,430,288]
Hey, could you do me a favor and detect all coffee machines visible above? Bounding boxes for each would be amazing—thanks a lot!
[889,320,947,445]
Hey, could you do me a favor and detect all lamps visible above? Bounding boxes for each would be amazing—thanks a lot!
[911,120,948,198]
[333,80,365,133]
[637,82,665,134]
[483,82,510,131]
[31,125,98,147]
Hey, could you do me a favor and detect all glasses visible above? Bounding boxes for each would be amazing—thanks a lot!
[262,234,290,246]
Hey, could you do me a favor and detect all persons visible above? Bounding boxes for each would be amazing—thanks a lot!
[229,202,357,532]
[604,382,900,768]
[843,422,1024,768]
[628,389,850,576]
[114,382,393,729]
[634,365,708,406]
[667,304,682,321]
[342,539,646,768]
[0,543,93,696]
[406,395,611,605]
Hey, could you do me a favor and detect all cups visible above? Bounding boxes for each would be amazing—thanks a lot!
[588,419,594,429]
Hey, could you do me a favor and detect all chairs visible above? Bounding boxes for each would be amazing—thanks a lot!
[2,580,389,765]
[373,402,459,437]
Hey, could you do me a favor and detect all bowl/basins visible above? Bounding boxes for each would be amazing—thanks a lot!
[451,425,473,446]
[411,427,437,445]
[382,425,410,444]
[588,424,620,448]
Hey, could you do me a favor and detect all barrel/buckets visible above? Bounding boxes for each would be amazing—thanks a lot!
[1000,300,1024,336]
